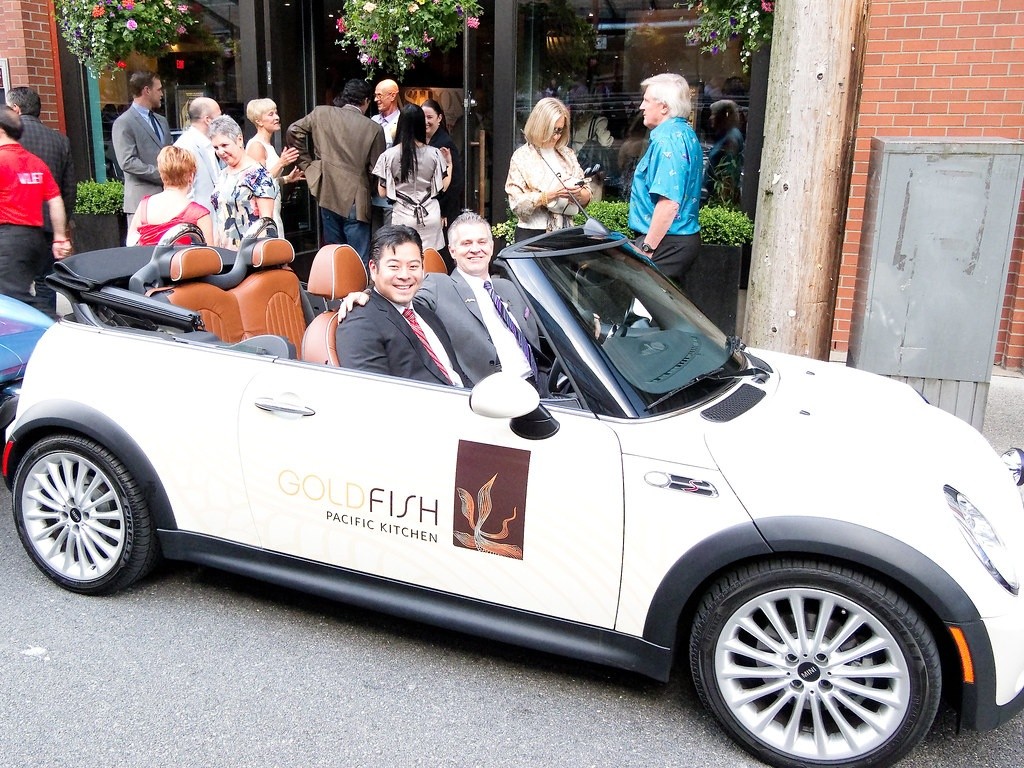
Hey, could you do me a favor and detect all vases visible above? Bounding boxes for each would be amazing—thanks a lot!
[681,245,743,342]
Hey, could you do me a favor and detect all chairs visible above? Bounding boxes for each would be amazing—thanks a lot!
[301,243,369,368]
[423,247,448,274]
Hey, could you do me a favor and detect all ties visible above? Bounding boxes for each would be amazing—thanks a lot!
[483,281,540,385]
[148,110,161,141]
[402,307,453,385]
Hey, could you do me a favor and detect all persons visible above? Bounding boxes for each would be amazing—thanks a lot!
[545,78,747,215]
[504,98,594,244]
[0,69,462,322]
[571,99,613,200]
[628,73,704,284]
[337,225,476,389]
[452,89,493,204]
[336,212,601,409]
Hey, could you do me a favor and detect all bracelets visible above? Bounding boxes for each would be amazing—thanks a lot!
[54,238,68,245]
[282,177,287,183]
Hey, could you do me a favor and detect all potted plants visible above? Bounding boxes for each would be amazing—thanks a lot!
[69,177,129,256]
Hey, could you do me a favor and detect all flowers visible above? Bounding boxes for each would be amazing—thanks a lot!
[203,39,241,61]
[491,199,754,247]
[672,0,775,74]
[50,0,195,81]
[333,0,484,82]
[533,3,601,92]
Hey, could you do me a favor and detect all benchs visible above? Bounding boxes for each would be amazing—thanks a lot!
[164,237,307,362]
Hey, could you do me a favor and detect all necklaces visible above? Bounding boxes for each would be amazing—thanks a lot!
[233,165,256,174]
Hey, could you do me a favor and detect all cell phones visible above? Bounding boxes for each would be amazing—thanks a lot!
[576,181,585,187]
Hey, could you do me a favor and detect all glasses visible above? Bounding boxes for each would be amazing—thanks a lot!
[551,127,565,134]
[373,92,396,100]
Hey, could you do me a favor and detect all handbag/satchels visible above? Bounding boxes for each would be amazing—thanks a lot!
[548,180,580,216]
[577,115,618,185]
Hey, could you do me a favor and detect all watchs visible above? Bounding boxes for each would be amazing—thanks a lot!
[642,239,657,254]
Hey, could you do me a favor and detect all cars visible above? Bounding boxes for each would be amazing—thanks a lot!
[0,222,1024,768]
[0,293,58,435]
